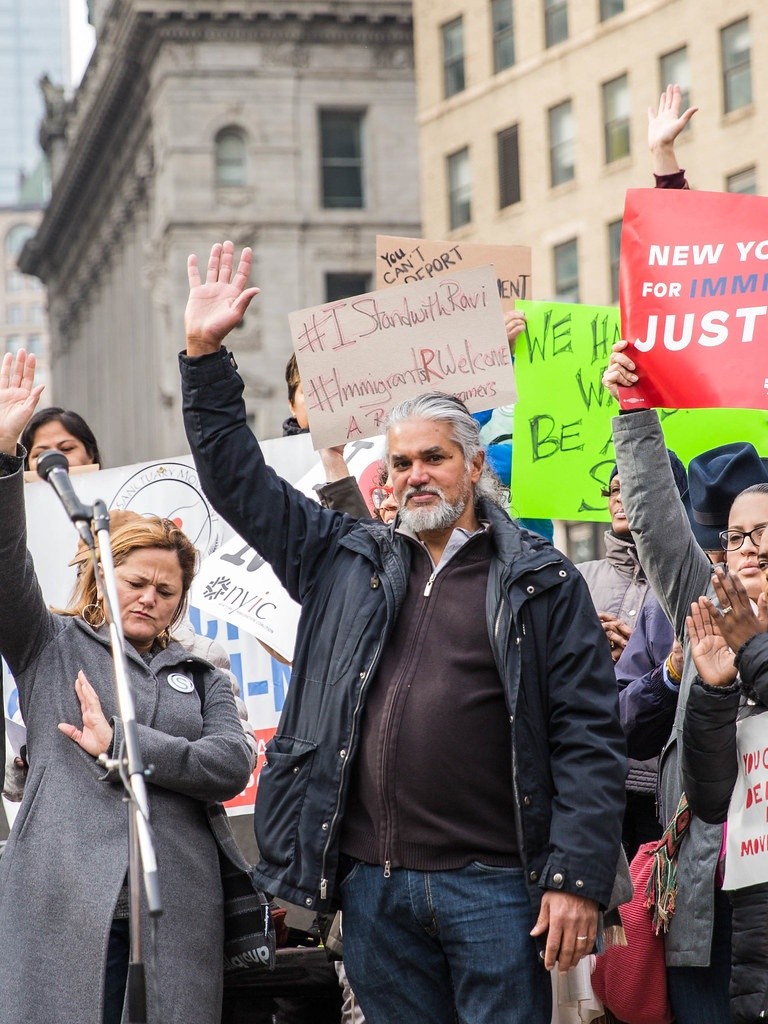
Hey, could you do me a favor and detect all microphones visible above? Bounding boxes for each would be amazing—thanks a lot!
[36,450,88,523]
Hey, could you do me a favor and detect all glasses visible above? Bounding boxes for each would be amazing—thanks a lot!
[718,525,766,551]
[369,486,399,509]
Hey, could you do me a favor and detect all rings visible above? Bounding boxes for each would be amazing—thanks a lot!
[576,936,588,941]
[722,606,732,614]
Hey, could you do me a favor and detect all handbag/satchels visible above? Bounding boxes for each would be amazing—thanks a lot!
[592,839,678,1024]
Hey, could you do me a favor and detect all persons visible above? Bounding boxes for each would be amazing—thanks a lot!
[570,448,690,865]
[20,404,102,472]
[314,306,555,549]
[0,350,255,1024]
[3,509,259,802]
[602,338,768,1024]
[282,351,312,438]
[176,239,628,1024]
[681,521,768,1024]
[614,441,767,762]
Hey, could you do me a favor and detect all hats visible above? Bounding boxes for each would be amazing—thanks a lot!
[609,448,689,508]
[684,441,768,551]
